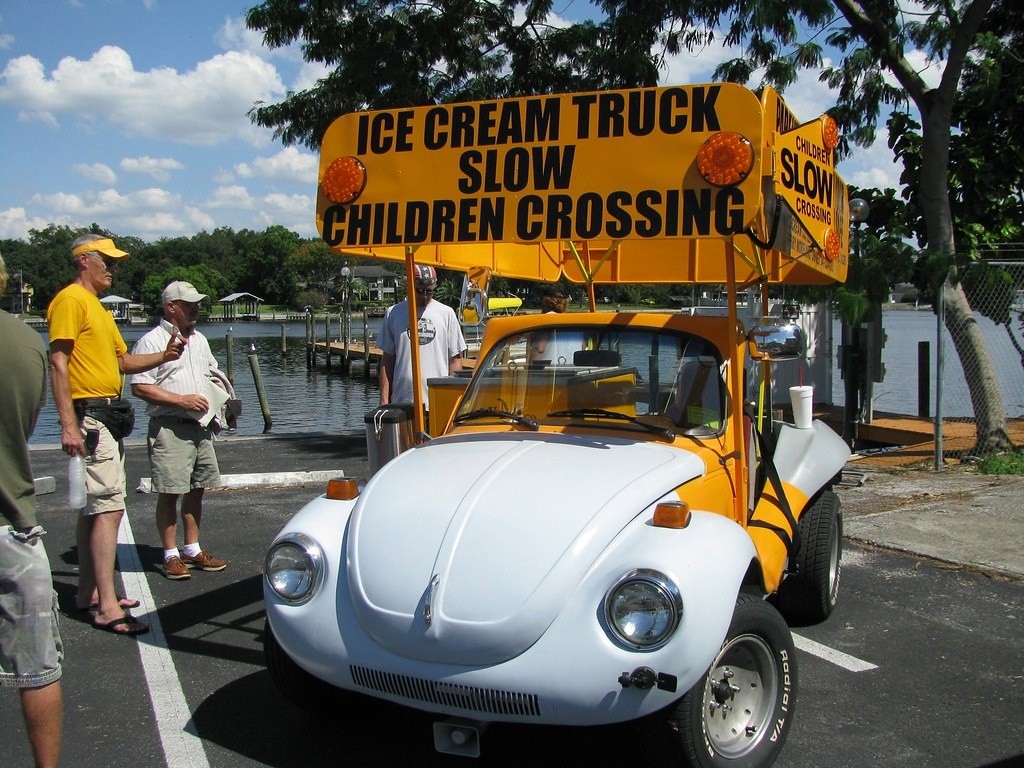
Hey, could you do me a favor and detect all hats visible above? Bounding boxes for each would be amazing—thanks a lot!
[162,280,210,305]
[411,263,437,289]
[72,239,130,260]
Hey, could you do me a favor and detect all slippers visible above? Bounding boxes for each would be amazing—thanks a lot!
[91,613,150,636]
[78,594,140,611]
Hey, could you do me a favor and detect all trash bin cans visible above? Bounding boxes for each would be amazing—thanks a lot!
[363,404,426,479]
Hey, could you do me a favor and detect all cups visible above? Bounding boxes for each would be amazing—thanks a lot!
[789,384,814,429]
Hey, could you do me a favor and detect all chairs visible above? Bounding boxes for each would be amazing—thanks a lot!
[676,360,721,430]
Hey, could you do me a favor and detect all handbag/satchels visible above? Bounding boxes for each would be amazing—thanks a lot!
[80,399,135,439]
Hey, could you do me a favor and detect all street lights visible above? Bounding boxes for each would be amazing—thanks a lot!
[339,266,358,350]
[848,199,871,425]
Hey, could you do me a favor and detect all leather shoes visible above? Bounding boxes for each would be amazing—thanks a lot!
[182,547,226,571]
[163,554,192,580]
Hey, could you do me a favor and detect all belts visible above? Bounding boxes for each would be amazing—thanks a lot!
[179,418,198,425]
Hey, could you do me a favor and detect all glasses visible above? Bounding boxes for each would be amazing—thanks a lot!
[416,288,437,294]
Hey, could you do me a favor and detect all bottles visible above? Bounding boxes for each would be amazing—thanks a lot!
[69,450,87,510]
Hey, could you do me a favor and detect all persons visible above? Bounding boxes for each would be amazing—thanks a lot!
[131,281,227,579]
[0,256,64,768]
[529,295,586,365]
[376,264,468,427]
[46,234,188,634]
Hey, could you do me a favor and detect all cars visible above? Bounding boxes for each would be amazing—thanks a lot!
[261,311,854,768]
